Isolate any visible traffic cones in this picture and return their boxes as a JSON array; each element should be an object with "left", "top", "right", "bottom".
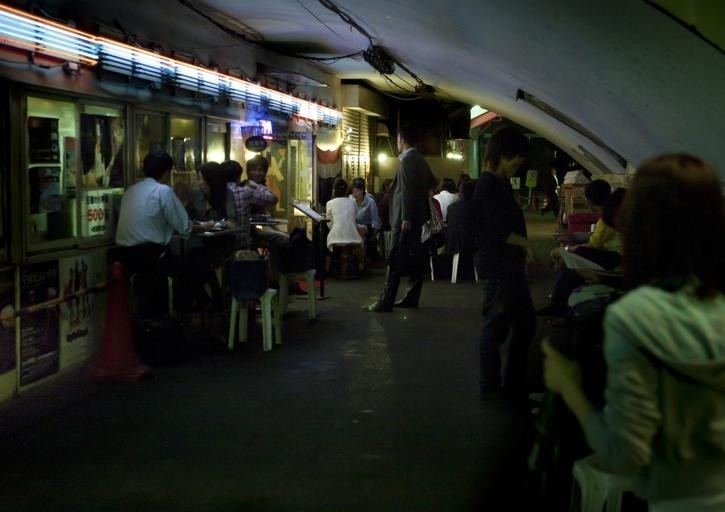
[{"left": 88, "top": 262, "right": 153, "bottom": 384}]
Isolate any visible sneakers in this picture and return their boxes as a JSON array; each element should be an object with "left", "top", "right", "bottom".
[
  {"left": 506, "top": 388, "right": 529, "bottom": 398},
  {"left": 480, "top": 387, "right": 502, "bottom": 397}
]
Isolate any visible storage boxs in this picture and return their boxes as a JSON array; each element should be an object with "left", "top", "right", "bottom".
[{"left": 561, "top": 170, "right": 591, "bottom": 215}]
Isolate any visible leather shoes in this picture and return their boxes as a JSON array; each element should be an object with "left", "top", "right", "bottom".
[
  {"left": 362, "top": 302, "right": 394, "bottom": 312},
  {"left": 394, "top": 299, "right": 418, "bottom": 309}
]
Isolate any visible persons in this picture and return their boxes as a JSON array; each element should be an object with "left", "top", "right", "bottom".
[
  {"left": 535, "top": 167, "right": 627, "bottom": 318},
  {"left": 319, "top": 171, "right": 475, "bottom": 279},
  {"left": 540, "top": 153, "right": 725, "bottom": 512},
  {"left": 367, "top": 124, "right": 437, "bottom": 312},
  {"left": 472, "top": 125, "right": 545, "bottom": 410},
  {"left": 64, "top": 260, "right": 92, "bottom": 328},
  {"left": 115, "top": 150, "right": 307, "bottom": 331}
]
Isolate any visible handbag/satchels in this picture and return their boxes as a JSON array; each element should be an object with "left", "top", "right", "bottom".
[{"left": 225, "top": 251, "right": 269, "bottom": 300}]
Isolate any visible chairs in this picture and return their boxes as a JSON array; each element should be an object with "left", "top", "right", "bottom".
[{"left": 448, "top": 214, "right": 480, "bottom": 284}]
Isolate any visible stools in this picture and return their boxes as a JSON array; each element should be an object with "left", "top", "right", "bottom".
[
  {"left": 570, "top": 453, "right": 635, "bottom": 512},
  {"left": 129, "top": 273, "right": 178, "bottom": 317},
  {"left": 333, "top": 243, "right": 361, "bottom": 280},
  {"left": 227, "top": 287, "right": 282, "bottom": 351},
  {"left": 421, "top": 240, "right": 438, "bottom": 282},
  {"left": 277, "top": 269, "right": 319, "bottom": 320}
]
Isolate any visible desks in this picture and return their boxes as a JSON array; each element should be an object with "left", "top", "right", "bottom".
[
  {"left": 195, "top": 227, "right": 237, "bottom": 329},
  {"left": 248, "top": 220, "right": 289, "bottom": 226}
]
